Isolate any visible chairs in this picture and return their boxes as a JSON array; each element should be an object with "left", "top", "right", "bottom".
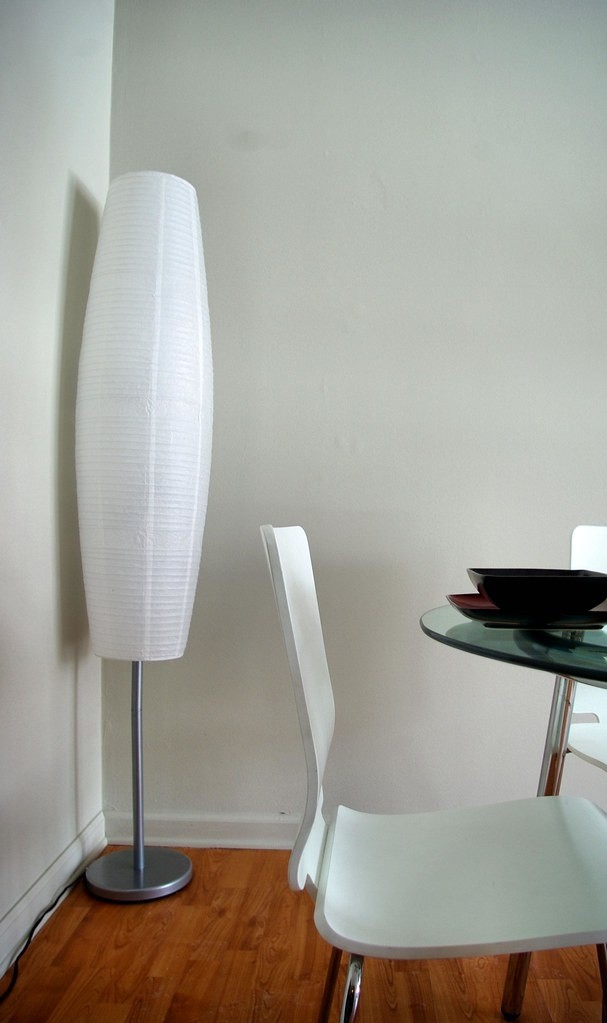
[
  {"left": 563, "top": 524, "right": 607, "bottom": 772},
  {"left": 256, "top": 520, "right": 607, "bottom": 1023}
]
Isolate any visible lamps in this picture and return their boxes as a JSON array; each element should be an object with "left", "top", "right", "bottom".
[{"left": 64, "top": 169, "right": 216, "bottom": 901}]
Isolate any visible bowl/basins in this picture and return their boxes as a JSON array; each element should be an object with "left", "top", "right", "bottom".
[{"left": 465, "top": 567, "right": 607, "bottom": 609}]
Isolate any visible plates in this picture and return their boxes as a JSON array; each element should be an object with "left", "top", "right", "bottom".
[{"left": 446, "top": 593, "right": 607, "bottom": 630}]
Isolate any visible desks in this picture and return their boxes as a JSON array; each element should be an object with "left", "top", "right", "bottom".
[{"left": 418, "top": 604, "right": 607, "bottom": 1023}]
